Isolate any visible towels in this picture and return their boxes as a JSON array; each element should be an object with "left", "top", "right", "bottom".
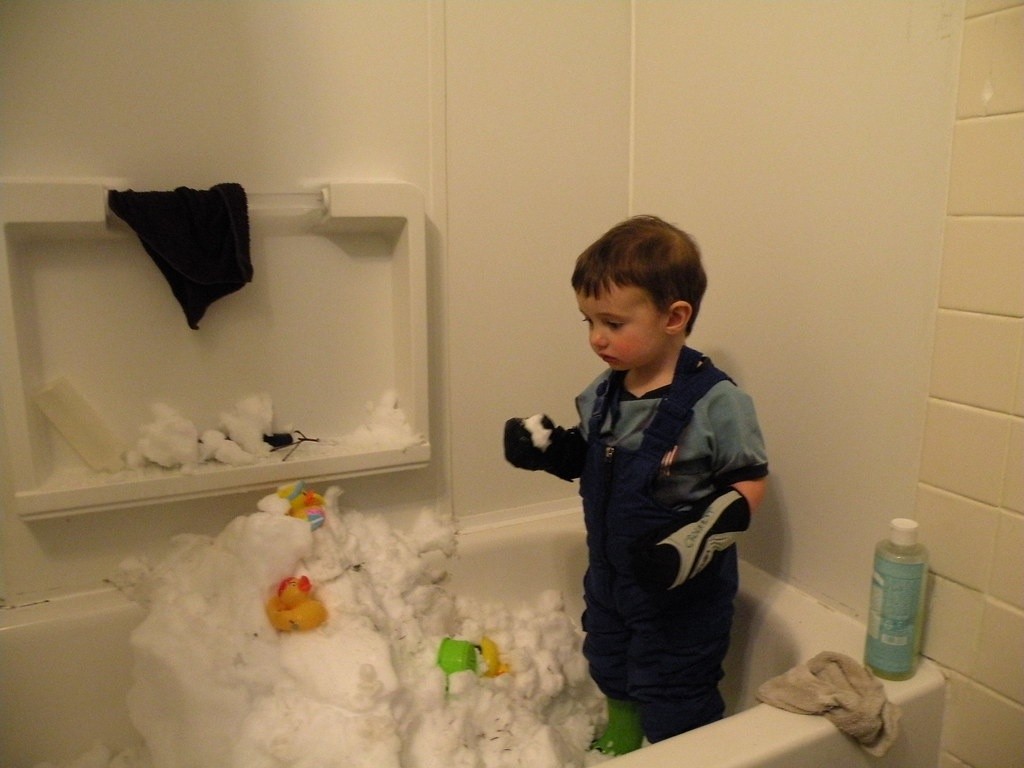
[
  {"left": 106, "top": 180, "right": 254, "bottom": 331},
  {"left": 752, "top": 648, "right": 903, "bottom": 759}
]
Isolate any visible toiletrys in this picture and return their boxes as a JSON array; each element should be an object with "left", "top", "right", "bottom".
[{"left": 862, "top": 516, "right": 933, "bottom": 684}]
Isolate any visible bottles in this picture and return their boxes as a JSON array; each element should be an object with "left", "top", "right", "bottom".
[
  {"left": 32, "top": 375, "right": 127, "bottom": 473},
  {"left": 863, "top": 517, "right": 929, "bottom": 681}
]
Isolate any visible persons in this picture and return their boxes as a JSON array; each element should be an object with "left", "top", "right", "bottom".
[{"left": 503, "top": 215, "right": 772, "bottom": 756}]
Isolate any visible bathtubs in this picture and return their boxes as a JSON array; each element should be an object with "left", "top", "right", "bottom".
[{"left": 0, "top": 491, "right": 951, "bottom": 768}]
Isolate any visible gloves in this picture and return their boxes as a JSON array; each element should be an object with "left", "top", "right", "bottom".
[
  {"left": 633, "top": 487, "right": 752, "bottom": 597},
  {"left": 504, "top": 414, "right": 584, "bottom": 483}
]
